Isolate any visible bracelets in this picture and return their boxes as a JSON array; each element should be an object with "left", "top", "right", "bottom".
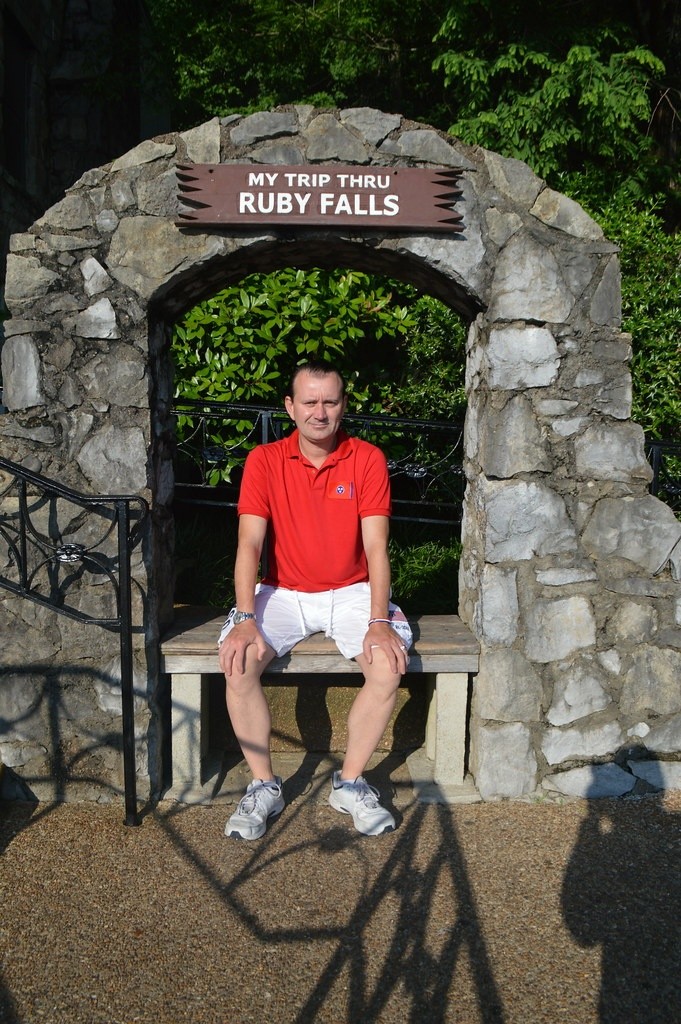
[{"left": 368, "top": 618, "right": 391, "bottom": 626}]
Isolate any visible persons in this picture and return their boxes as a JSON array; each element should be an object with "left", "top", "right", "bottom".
[{"left": 216, "top": 358, "right": 413, "bottom": 842}]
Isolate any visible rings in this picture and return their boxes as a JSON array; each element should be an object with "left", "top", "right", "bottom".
[{"left": 400, "top": 644, "right": 407, "bottom": 651}]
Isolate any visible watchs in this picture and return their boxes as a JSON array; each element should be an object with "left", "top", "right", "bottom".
[{"left": 233, "top": 611, "right": 257, "bottom": 626}]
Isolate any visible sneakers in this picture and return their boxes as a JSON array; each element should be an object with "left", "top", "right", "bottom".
[
  {"left": 224, "top": 776, "right": 285, "bottom": 841},
  {"left": 329, "top": 769, "right": 396, "bottom": 836}
]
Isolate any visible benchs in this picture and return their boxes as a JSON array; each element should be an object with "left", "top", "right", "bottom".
[{"left": 158, "top": 603, "right": 484, "bottom": 806}]
